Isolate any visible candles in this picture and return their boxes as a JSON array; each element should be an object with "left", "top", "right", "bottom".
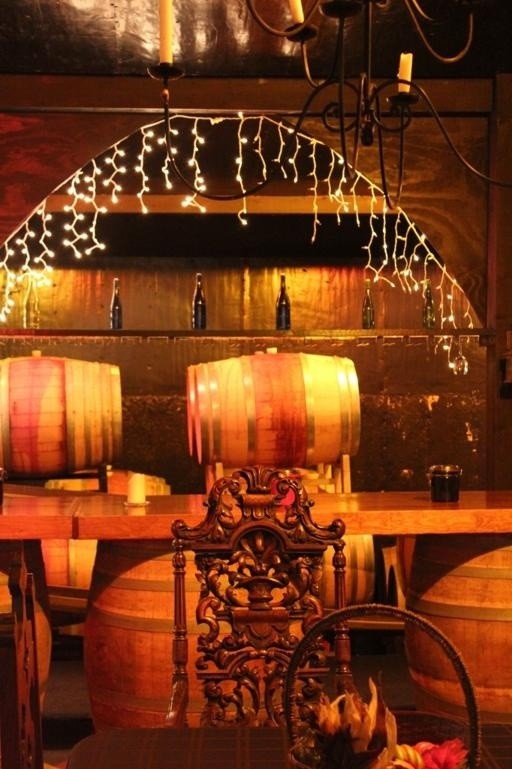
[
  {"left": 397, "top": 52, "right": 414, "bottom": 94},
  {"left": 288, "top": 0, "right": 304, "bottom": 24},
  {"left": 158, "top": 0, "right": 173, "bottom": 67}
]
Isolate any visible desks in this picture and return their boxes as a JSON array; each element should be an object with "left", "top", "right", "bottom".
[{"left": 2, "top": 487, "right": 512, "bottom": 769}]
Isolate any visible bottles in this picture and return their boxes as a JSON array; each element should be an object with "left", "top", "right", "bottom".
[
  {"left": 423, "top": 279, "right": 436, "bottom": 330},
  {"left": 429, "top": 466, "right": 460, "bottom": 501},
  {"left": 110, "top": 279, "right": 122, "bottom": 330},
  {"left": 362, "top": 279, "right": 374, "bottom": 329},
  {"left": 23, "top": 275, "right": 39, "bottom": 329},
  {"left": 192, "top": 274, "right": 206, "bottom": 330},
  {"left": 275, "top": 275, "right": 290, "bottom": 330}
]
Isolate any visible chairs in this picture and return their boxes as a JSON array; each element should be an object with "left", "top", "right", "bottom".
[{"left": 166, "top": 465, "right": 354, "bottom": 731}]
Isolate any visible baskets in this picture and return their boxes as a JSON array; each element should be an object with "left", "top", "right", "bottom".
[{"left": 284, "top": 604, "right": 482, "bottom": 769}]
